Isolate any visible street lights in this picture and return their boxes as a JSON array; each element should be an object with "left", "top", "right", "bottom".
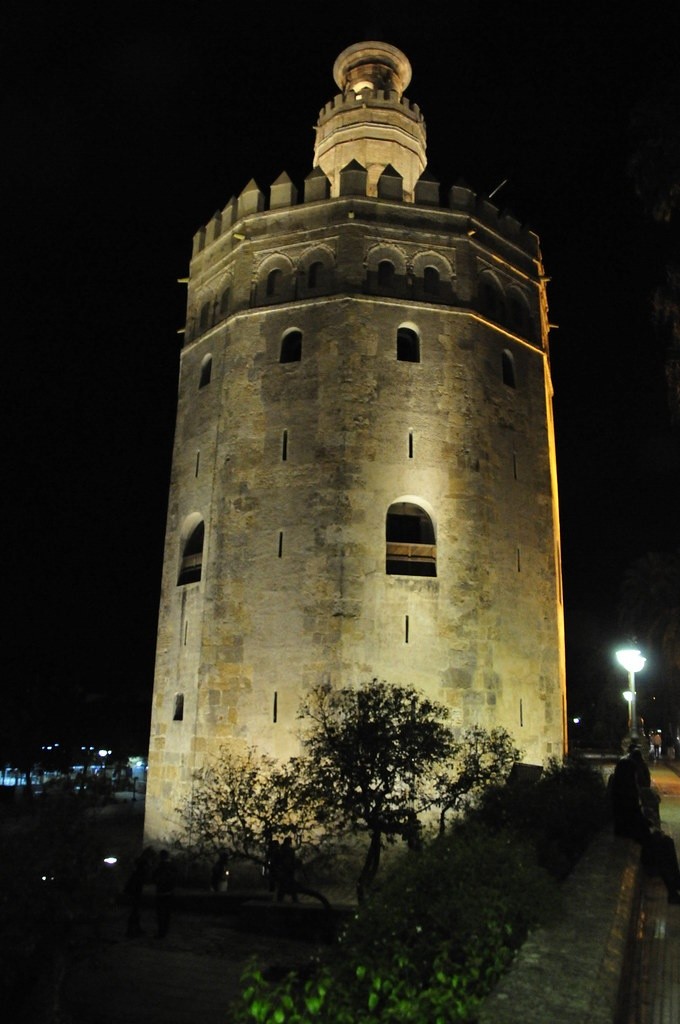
[
  {"left": 80, "top": 746, "right": 95, "bottom": 776},
  {"left": 615, "top": 646, "right": 647, "bottom": 754},
  {"left": 99, "top": 749, "right": 112, "bottom": 778}
]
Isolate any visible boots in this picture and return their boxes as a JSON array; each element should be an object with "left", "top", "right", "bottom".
[{"left": 667, "top": 888, "right": 680, "bottom": 904}]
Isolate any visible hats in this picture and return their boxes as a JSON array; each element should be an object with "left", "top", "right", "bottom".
[{"left": 618, "top": 760, "right": 638, "bottom": 769}]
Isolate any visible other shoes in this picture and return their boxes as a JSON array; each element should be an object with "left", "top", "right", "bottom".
[
  {"left": 123, "top": 926, "right": 146, "bottom": 935},
  {"left": 154, "top": 930, "right": 165, "bottom": 937}
]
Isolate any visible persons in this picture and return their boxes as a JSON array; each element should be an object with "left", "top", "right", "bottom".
[
  {"left": 204, "top": 853, "right": 229, "bottom": 890},
  {"left": 124, "top": 846, "right": 176, "bottom": 939},
  {"left": 266, "top": 837, "right": 302, "bottom": 902},
  {"left": 607, "top": 751, "right": 680, "bottom": 904}
]
